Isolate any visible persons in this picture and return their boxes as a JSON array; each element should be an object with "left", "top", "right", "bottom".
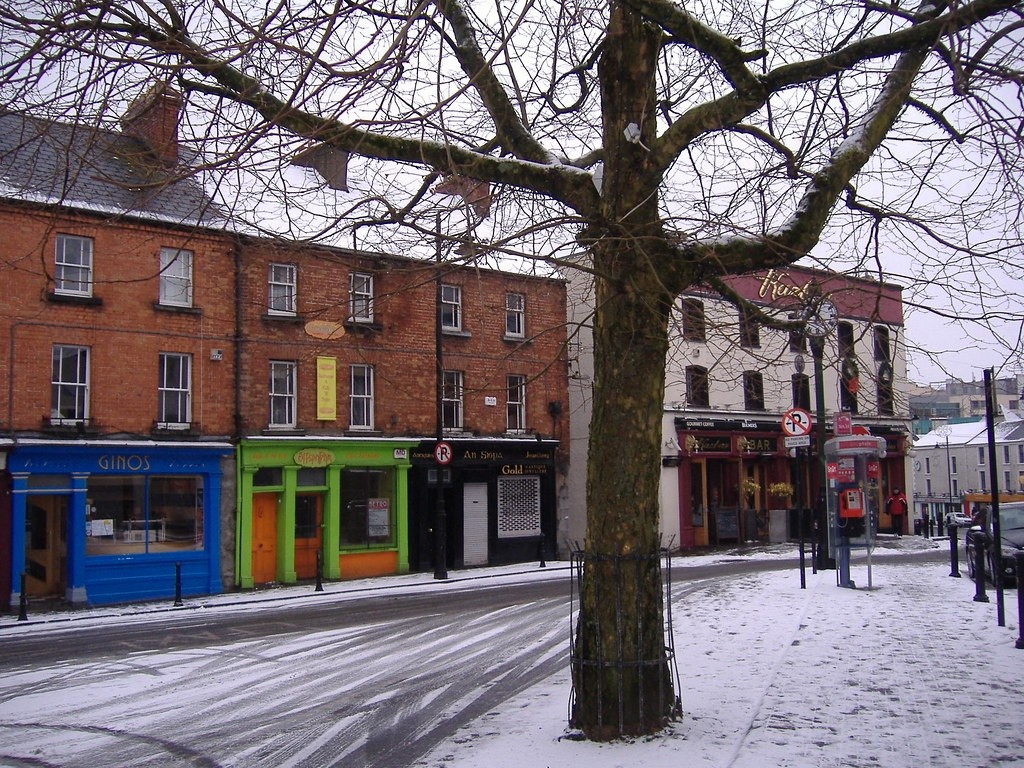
[
  {"left": 885, "top": 486, "right": 908, "bottom": 538},
  {"left": 972, "top": 506, "right": 977, "bottom": 515}
]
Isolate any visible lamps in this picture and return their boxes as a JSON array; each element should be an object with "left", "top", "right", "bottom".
[
  {"left": 746, "top": 442, "right": 751, "bottom": 455},
  {"left": 903, "top": 440, "right": 916, "bottom": 458},
  {"left": 461, "top": 430, "right": 481, "bottom": 438},
  {"left": 784, "top": 440, "right": 796, "bottom": 457},
  {"left": 522, "top": 433, "right": 542, "bottom": 441},
  {"left": 693, "top": 441, "right": 699, "bottom": 453}
]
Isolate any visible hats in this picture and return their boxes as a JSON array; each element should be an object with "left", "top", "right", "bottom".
[{"left": 893, "top": 486, "right": 899, "bottom": 490}]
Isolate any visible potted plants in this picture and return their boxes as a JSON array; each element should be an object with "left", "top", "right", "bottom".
[
  {"left": 768, "top": 484, "right": 792, "bottom": 510},
  {"left": 739, "top": 478, "right": 761, "bottom": 540}
]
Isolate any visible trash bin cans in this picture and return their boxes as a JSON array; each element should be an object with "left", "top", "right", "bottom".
[
  {"left": 947, "top": 523, "right": 956, "bottom": 536},
  {"left": 914, "top": 519, "right": 921, "bottom": 536}
]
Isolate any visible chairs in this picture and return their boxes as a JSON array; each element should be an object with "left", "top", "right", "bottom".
[{"left": 999, "top": 515, "right": 1017, "bottom": 529}]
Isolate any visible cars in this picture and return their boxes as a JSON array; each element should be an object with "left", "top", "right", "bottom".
[
  {"left": 943, "top": 512, "right": 973, "bottom": 528},
  {"left": 965, "top": 501, "right": 1024, "bottom": 587}
]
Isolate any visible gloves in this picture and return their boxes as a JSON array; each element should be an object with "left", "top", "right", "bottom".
[
  {"left": 905, "top": 509, "right": 908, "bottom": 516},
  {"left": 885, "top": 510, "right": 889, "bottom": 515}
]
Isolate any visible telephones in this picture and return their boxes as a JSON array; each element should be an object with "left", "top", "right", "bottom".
[{"left": 839, "top": 489, "right": 863, "bottom": 517}]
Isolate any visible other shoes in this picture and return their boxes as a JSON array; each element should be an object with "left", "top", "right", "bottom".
[
  {"left": 894, "top": 533, "right": 897, "bottom": 537},
  {"left": 898, "top": 535, "right": 902, "bottom": 538}
]
